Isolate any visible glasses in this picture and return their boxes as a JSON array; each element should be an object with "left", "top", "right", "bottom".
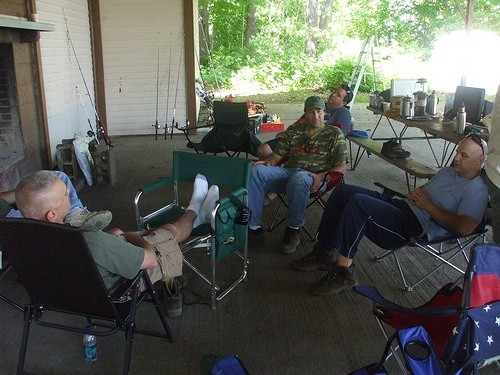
[
  {"left": 340, "top": 84, "right": 351, "bottom": 98},
  {"left": 303, "top": 135, "right": 310, "bottom": 152},
  {"left": 466, "top": 134, "right": 484, "bottom": 155},
  {"left": 55, "top": 184, "right": 70, "bottom": 208}
]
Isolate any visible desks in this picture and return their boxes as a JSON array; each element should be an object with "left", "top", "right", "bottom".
[{"left": 366, "top": 106, "right": 490, "bottom": 168}]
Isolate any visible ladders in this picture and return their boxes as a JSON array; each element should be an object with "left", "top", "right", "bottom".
[{"left": 348, "top": 36, "right": 385, "bottom": 116}]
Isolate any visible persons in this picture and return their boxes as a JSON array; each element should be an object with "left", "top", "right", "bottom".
[
  {"left": 248, "top": 96, "right": 350, "bottom": 254},
  {"left": 0, "top": 171, "right": 219, "bottom": 303},
  {"left": 292, "top": 133, "right": 488, "bottom": 296},
  {"left": 258, "top": 84, "right": 354, "bottom": 208}
]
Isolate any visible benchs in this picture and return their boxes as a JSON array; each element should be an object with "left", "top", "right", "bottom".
[{"left": 346, "top": 134, "right": 437, "bottom": 194}]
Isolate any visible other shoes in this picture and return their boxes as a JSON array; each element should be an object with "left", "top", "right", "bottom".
[{"left": 381, "top": 138, "right": 411, "bottom": 159}]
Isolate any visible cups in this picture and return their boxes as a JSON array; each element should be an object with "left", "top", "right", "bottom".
[{"left": 382, "top": 102, "right": 391, "bottom": 112}]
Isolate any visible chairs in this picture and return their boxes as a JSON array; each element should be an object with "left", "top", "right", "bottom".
[
  {"left": 133, "top": 151, "right": 252, "bottom": 311},
  {"left": 266, "top": 119, "right": 345, "bottom": 243},
  {"left": 185, "top": 101, "right": 263, "bottom": 158},
  {"left": 0, "top": 216, "right": 174, "bottom": 375},
  {"left": 371, "top": 165, "right": 500, "bottom": 293},
  {"left": 352, "top": 242, "right": 500, "bottom": 375}
]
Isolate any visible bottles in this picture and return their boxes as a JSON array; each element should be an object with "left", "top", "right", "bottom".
[
  {"left": 82, "top": 325, "right": 97, "bottom": 362},
  {"left": 349, "top": 117, "right": 354, "bottom": 133},
  {"left": 400, "top": 92, "right": 427, "bottom": 116}
]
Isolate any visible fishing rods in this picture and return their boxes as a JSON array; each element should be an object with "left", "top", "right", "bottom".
[
  {"left": 162, "top": 32, "right": 171, "bottom": 139},
  {"left": 170, "top": 31, "right": 184, "bottom": 140},
  {"left": 62, "top": 8, "right": 112, "bottom": 147},
  {"left": 76, "top": 85, "right": 100, "bottom": 145},
  {"left": 152, "top": 32, "right": 161, "bottom": 140}
]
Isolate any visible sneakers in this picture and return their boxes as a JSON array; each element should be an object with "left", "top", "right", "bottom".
[
  {"left": 279, "top": 226, "right": 301, "bottom": 253},
  {"left": 309, "top": 260, "right": 359, "bottom": 296},
  {"left": 248, "top": 226, "right": 265, "bottom": 244},
  {"left": 291, "top": 243, "right": 337, "bottom": 272}
]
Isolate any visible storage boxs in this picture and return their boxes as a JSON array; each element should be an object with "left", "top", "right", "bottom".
[
  {"left": 247, "top": 113, "right": 268, "bottom": 135},
  {"left": 262, "top": 120, "right": 284, "bottom": 131}
]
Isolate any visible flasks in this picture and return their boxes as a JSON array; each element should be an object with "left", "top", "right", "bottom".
[
  {"left": 430, "top": 90, "right": 437, "bottom": 115},
  {"left": 458, "top": 106, "right": 466, "bottom": 135}
]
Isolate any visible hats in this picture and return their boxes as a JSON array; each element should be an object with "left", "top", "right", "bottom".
[{"left": 305, "top": 96, "right": 325, "bottom": 110}]
